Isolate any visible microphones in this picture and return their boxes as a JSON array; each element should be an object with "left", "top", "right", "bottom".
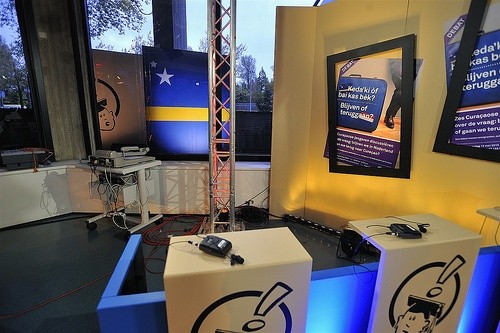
[
  {"left": 230, "top": 254, "right": 244, "bottom": 265},
  {"left": 418, "top": 225, "right": 427, "bottom": 233}
]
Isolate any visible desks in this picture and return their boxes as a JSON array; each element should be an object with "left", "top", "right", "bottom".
[
  {"left": 75, "top": 160, "right": 164, "bottom": 242},
  {"left": 97, "top": 206, "right": 500, "bottom": 333}
]
